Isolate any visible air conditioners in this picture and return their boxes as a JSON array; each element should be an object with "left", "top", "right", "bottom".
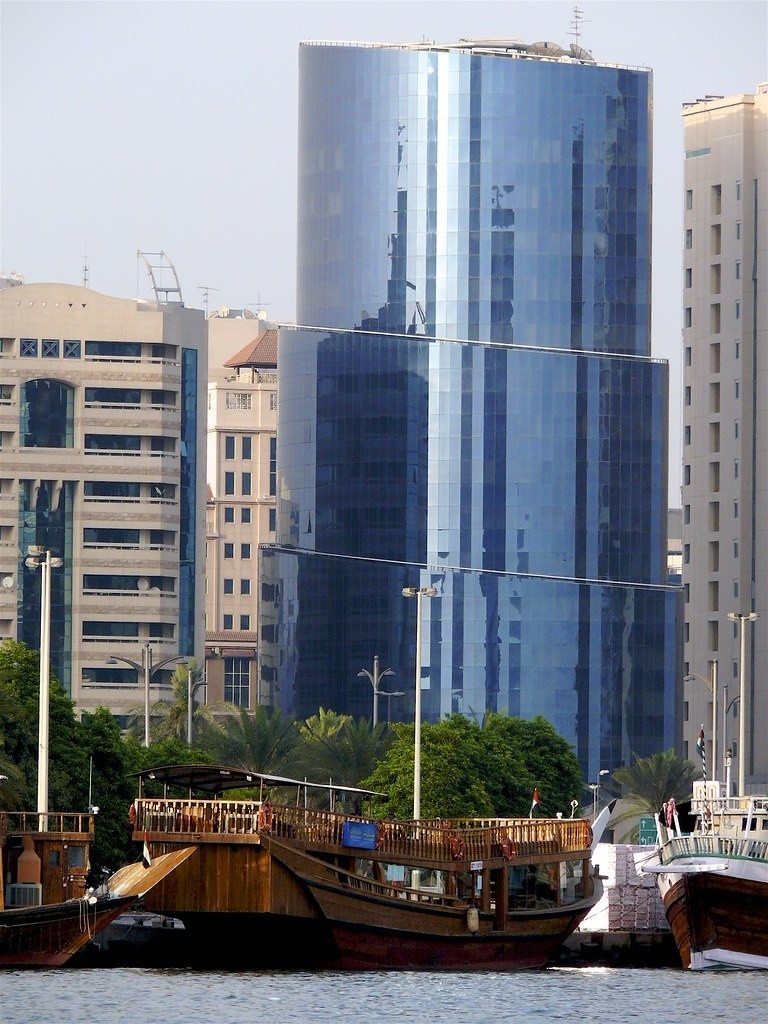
[{"left": 5, "top": 883, "right": 42, "bottom": 909}]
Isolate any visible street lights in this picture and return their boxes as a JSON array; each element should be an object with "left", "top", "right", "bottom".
[
  {"left": 726, "top": 611, "right": 761, "bottom": 798},
  {"left": 374, "top": 691, "right": 405, "bottom": 724},
  {"left": 357, "top": 655, "right": 398, "bottom": 729},
  {"left": 402, "top": 586, "right": 438, "bottom": 838},
  {"left": 24, "top": 544, "right": 64, "bottom": 833},
  {"left": 105, "top": 642, "right": 190, "bottom": 749},
  {"left": 684, "top": 658, "right": 720, "bottom": 781}
]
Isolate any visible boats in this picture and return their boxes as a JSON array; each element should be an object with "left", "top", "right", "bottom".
[
  {"left": 0, "top": 801, "right": 106, "bottom": 973},
  {"left": 131, "top": 788, "right": 605, "bottom": 976},
  {"left": 641, "top": 722, "right": 768, "bottom": 977}
]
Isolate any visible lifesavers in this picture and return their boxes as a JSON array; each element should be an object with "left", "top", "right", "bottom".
[
  {"left": 373, "top": 822, "right": 386, "bottom": 849},
  {"left": 129, "top": 803, "right": 135, "bottom": 826},
  {"left": 441, "top": 818, "right": 453, "bottom": 837},
  {"left": 502, "top": 837, "right": 516, "bottom": 861},
  {"left": 451, "top": 837, "right": 465, "bottom": 860},
  {"left": 583, "top": 825, "right": 593, "bottom": 849},
  {"left": 259, "top": 805, "right": 272, "bottom": 834}
]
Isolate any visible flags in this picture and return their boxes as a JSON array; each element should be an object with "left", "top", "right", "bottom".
[
  {"left": 529, "top": 788, "right": 541, "bottom": 819},
  {"left": 696, "top": 724, "right": 705, "bottom": 759},
  {"left": 142, "top": 828, "right": 151, "bottom": 869}
]
